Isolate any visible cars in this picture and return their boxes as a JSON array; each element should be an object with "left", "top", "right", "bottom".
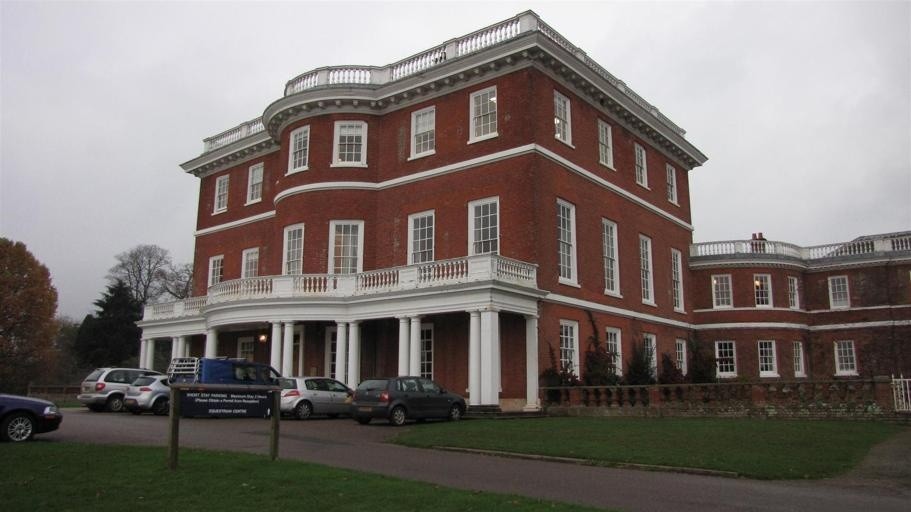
[{"left": 0, "top": 394, "right": 61, "bottom": 441}]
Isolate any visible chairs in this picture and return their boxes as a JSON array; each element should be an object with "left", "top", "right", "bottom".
[{"left": 406, "top": 382, "right": 416, "bottom": 390}]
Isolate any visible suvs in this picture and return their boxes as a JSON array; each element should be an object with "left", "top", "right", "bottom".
[
  {"left": 351, "top": 376, "right": 466, "bottom": 425},
  {"left": 275, "top": 377, "right": 351, "bottom": 420},
  {"left": 167, "top": 354, "right": 283, "bottom": 384},
  {"left": 77, "top": 367, "right": 171, "bottom": 416}
]
